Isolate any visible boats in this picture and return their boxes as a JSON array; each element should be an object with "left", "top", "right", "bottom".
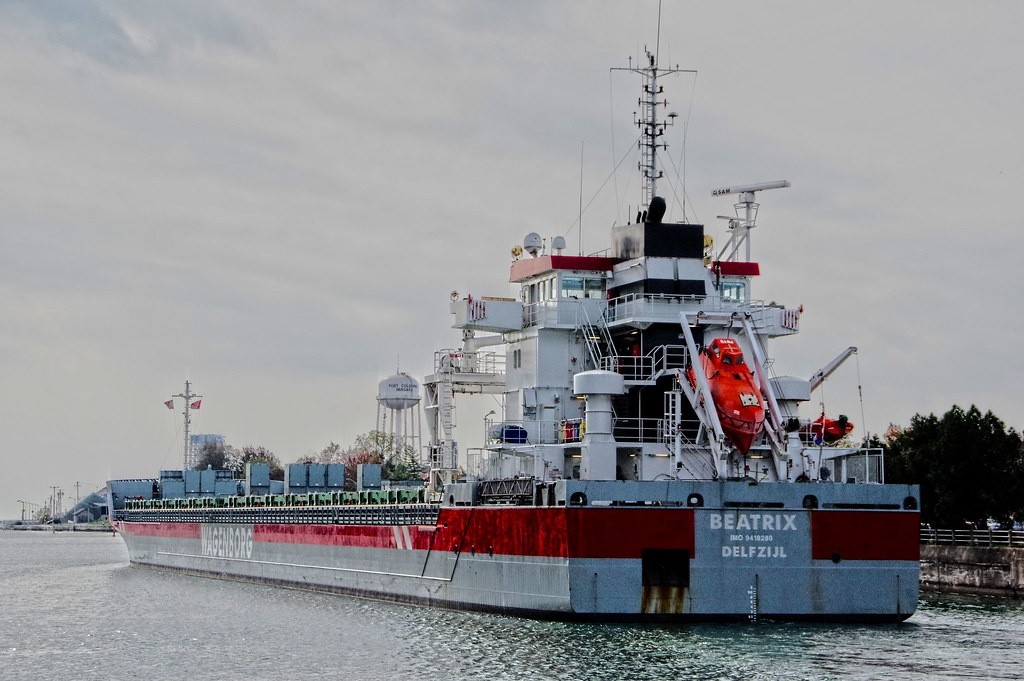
[
  {"left": 800, "top": 413, "right": 855, "bottom": 442},
  {"left": 11, "top": 523, "right": 115, "bottom": 530},
  {"left": 685, "top": 338, "right": 765, "bottom": 457},
  {"left": 107, "top": 0, "right": 921, "bottom": 620}
]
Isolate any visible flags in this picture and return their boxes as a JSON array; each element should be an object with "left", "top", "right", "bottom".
[
  {"left": 191, "top": 400, "right": 201, "bottom": 408},
  {"left": 165, "top": 400, "right": 174, "bottom": 409}
]
